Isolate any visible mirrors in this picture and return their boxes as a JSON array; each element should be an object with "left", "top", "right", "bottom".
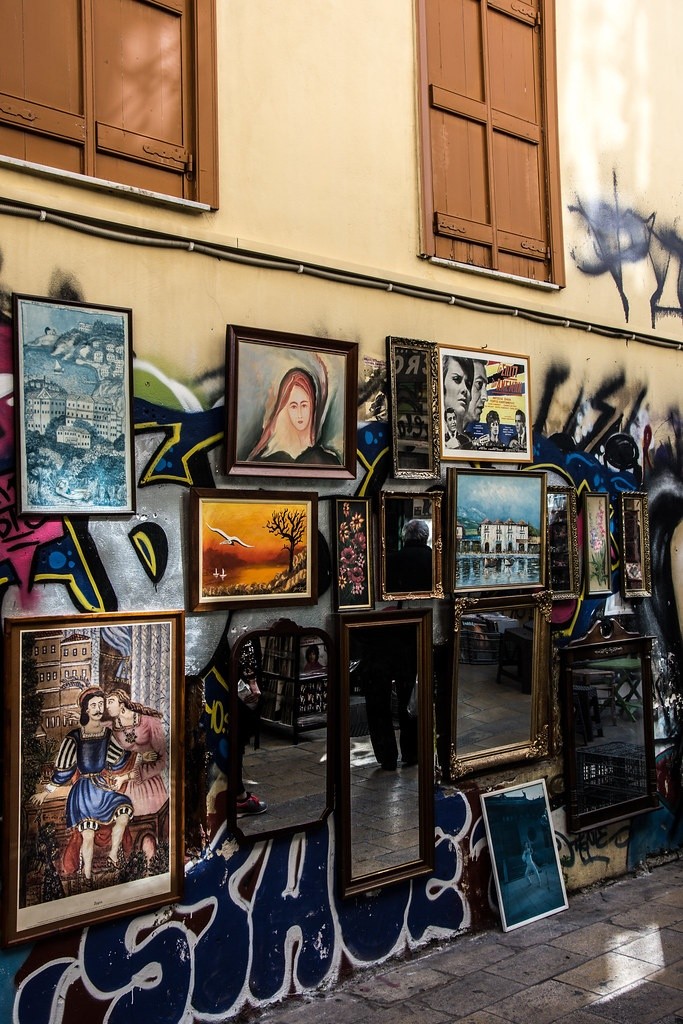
[
  {"left": 547, "top": 485, "right": 581, "bottom": 601},
  {"left": 559, "top": 618, "right": 664, "bottom": 837},
  {"left": 449, "top": 589, "right": 554, "bottom": 782},
  {"left": 385, "top": 335, "right": 442, "bottom": 481},
  {"left": 228, "top": 616, "right": 334, "bottom": 845},
  {"left": 333, "top": 608, "right": 435, "bottom": 901},
  {"left": 379, "top": 490, "right": 445, "bottom": 601}
]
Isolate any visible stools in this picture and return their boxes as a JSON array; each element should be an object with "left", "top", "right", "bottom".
[
  {"left": 572, "top": 684, "right": 603, "bottom": 741},
  {"left": 588, "top": 659, "right": 641, "bottom": 723},
  {"left": 572, "top": 668, "right": 617, "bottom": 727}
]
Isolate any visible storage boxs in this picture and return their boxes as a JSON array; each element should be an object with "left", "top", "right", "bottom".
[{"left": 575, "top": 739, "right": 646, "bottom": 815}]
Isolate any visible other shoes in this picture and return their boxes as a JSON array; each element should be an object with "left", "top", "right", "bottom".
[
  {"left": 381, "top": 760, "right": 397, "bottom": 771},
  {"left": 401, "top": 754, "right": 417, "bottom": 764}
]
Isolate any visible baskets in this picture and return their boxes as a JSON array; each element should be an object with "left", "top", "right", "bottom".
[
  {"left": 459, "top": 629, "right": 501, "bottom": 665},
  {"left": 576, "top": 740, "right": 648, "bottom": 814}
]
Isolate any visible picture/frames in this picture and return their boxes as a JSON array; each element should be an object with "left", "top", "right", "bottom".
[
  {"left": 436, "top": 342, "right": 534, "bottom": 464},
  {"left": 584, "top": 491, "right": 612, "bottom": 597},
  {"left": 226, "top": 323, "right": 359, "bottom": 479},
  {"left": 480, "top": 778, "right": 569, "bottom": 933},
  {"left": 330, "top": 494, "right": 376, "bottom": 612},
  {"left": 11, "top": 291, "right": 136, "bottom": 516},
  {"left": 188, "top": 486, "right": 318, "bottom": 613},
  {"left": 618, "top": 491, "right": 652, "bottom": 599},
  {"left": 0, "top": 609, "right": 188, "bottom": 949},
  {"left": 445, "top": 465, "right": 545, "bottom": 593}
]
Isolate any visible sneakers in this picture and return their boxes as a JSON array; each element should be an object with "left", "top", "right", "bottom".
[{"left": 237, "top": 791, "right": 269, "bottom": 818}]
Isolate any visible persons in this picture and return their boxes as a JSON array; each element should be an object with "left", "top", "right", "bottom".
[
  {"left": 353, "top": 623, "right": 419, "bottom": 769},
  {"left": 388, "top": 519, "right": 432, "bottom": 590},
  {"left": 443, "top": 356, "right": 526, "bottom": 453},
  {"left": 234, "top": 637, "right": 268, "bottom": 818},
  {"left": 521, "top": 841, "right": 542, "bottom": 887}
]
